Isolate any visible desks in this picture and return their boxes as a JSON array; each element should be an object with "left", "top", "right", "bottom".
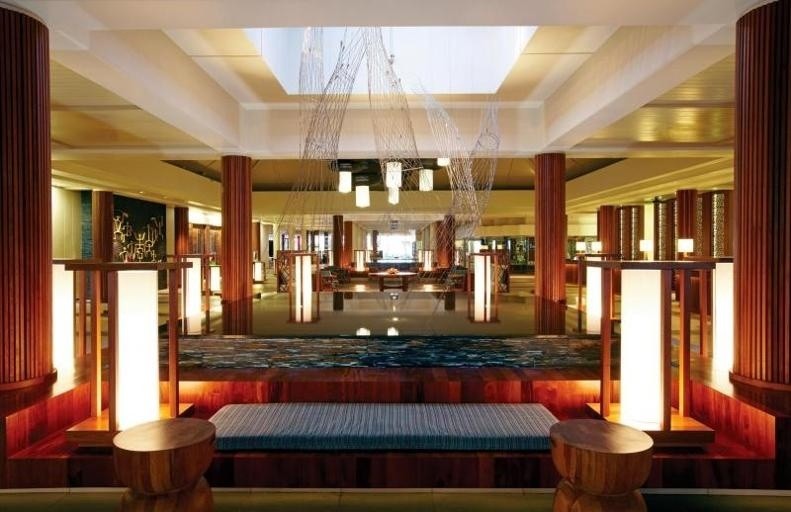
[{"left": 368, "top": 272, "right": 419, "bottom": 292}]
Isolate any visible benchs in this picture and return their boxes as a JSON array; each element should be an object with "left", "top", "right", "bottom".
[{"left": 204, "top": 402, "right": 560, "bottom": 486}]
